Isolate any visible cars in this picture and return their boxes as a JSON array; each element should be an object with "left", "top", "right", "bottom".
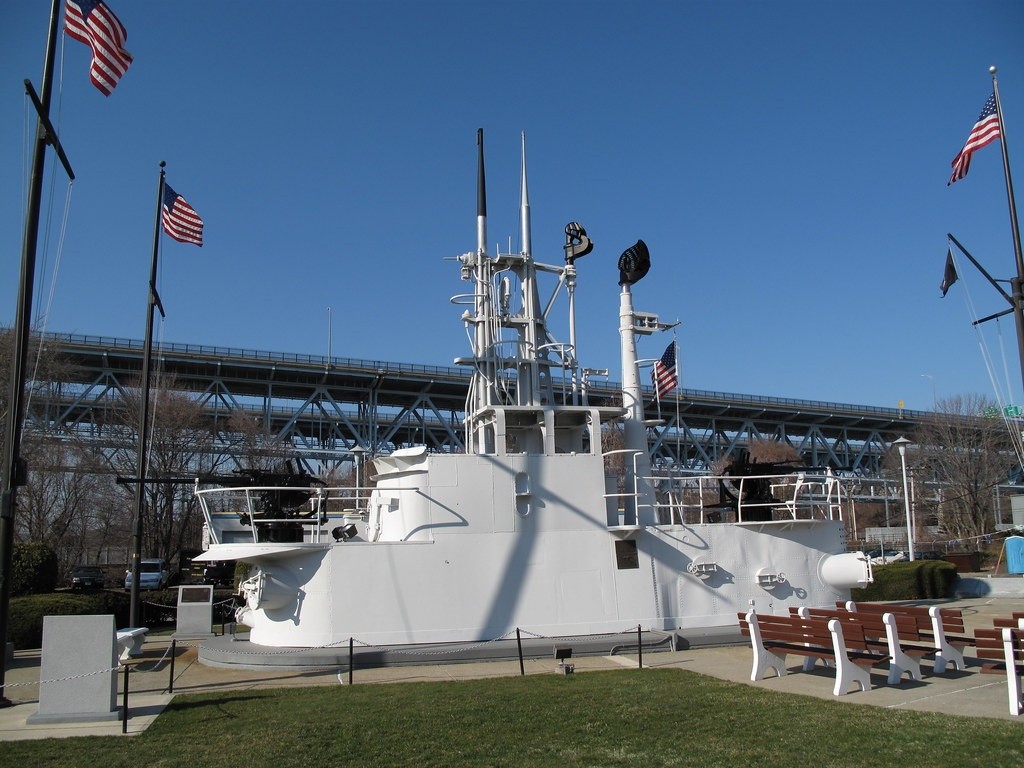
[{"left": 72, "top": 565, "right": 106, "bottom": 591}]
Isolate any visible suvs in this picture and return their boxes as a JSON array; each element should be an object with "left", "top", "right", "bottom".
[{"left": 125, "top": 558, "right": 169, "bottom": 592}]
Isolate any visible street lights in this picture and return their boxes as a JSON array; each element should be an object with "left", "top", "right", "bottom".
[
  {"left": 892, "top": 436, "right": 914, "bottom": 562},
  {"left": 921, "top": 374, "right": 937, "bottom": 423}
]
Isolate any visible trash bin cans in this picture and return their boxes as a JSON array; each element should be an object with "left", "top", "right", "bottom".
[{"left": 1005, "top": 536, "right": 1024, "bottom": 575}]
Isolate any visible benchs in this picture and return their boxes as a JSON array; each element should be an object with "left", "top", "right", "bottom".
[
  {"left": 117, "top": 627, "right": 149, "bottom": 666},
  {"left": 736, "top": 600, "right": 1024, "bottom": 716}
]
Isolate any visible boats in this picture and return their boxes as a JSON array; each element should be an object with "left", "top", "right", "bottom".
[{"left": 192, "top": 127, "right": 873, "bottom": 670}]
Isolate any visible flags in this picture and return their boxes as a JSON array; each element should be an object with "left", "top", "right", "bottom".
[
  {"left": 162, "top": 182, "right": 204, "bottom": 248},
  {"left": 650, "top": 342, "right": 678, "bottom": 402},
  {"left": 947, "top": 91, "right": 1001, "bottom": 186},
  {"left": 940, "top": 250, "right": 958, "bottom": 298},
  {"left": 64, "top": 0, "right": 135, "bottom": 97}
]
